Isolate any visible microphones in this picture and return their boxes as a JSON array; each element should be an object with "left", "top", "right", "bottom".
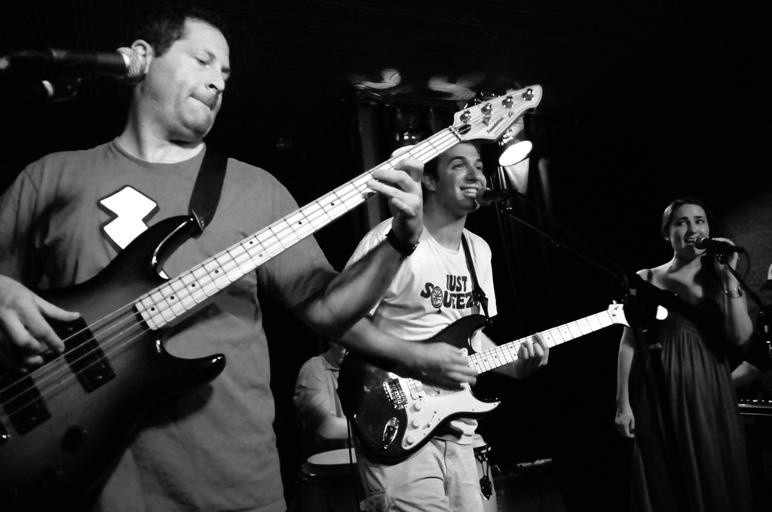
[
  {"left": 44, "top": 45, "right": 148, "bottom": 86},
  {"left": 475, "top": 187, "right": 509, "bottom": 206},
  {"left": 693, "top": 233, "right": 746, "bottom": 255}
]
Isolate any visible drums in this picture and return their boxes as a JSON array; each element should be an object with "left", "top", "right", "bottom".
[{"left": 298, "top": 447, "right": 366, "bottom": 512}]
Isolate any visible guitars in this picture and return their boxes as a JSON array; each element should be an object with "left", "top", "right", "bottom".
[
  {"left": 339, "top": 299, "right": 631, "bottom": 464},
  {"left": 0, "top": 83, "right": 544, "bottom": 512}
]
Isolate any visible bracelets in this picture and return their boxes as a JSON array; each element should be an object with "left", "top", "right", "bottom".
[
  {"left": 385, "top": 227, "right": 419, "bottom": 258},
  {"left": 723, "top": 286, "right": 745, "bottom": 301}
]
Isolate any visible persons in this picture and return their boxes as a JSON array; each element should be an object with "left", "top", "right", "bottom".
[
  {"left": 328, "top": 138, "right": 551, "bottom": 512},
  {"left": 614, "top": 195, "right": 761, "bottom": 510},
  {"left": 0, "top": 6, "right": 424, "bottom": 512},
  {"left": 291, "top": 325, "right": 355, "bottom": 442}
]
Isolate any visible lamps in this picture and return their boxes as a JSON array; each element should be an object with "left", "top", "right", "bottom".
[{"left": 498, "top": 137, "right": 533, "bottom": 167}]
